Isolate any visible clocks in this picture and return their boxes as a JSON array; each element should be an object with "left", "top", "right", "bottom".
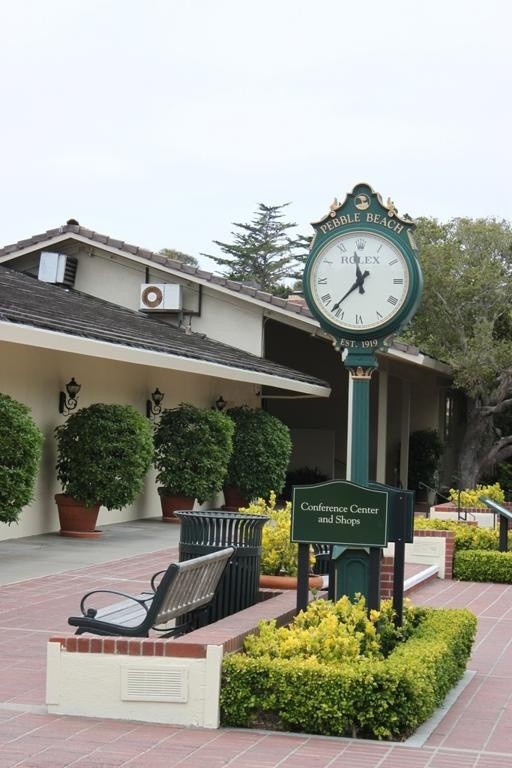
[{"left": 309, "top": 231, "right": 410, "bottom": 331}]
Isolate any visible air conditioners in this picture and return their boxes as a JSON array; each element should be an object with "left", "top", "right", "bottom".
[{"left": 139, "top": 283, "right": 183, "bottom": 312}]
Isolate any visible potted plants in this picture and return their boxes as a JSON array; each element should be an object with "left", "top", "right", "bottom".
[
  {"left": 53, "top": 403, "right": 155, "bottom": 531},
  {"left": 152, "top": 402, "right": 237, "bottom": 517},
  {"left": 223, "top": 403, "right": 294, "bottom": 507}
]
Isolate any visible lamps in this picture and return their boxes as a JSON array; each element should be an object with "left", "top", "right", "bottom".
[{"left": 59, "top": 377, "right": 227, "bottom": 421}]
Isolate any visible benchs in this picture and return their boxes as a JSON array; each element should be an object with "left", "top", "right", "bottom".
[{"left": 68, "top": 546, "right": 238, "bottom": 639}]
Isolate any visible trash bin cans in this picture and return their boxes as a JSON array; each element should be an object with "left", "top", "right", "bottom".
[{"left": 171, "top": 509, "right": 271, "bottom": 639}]
